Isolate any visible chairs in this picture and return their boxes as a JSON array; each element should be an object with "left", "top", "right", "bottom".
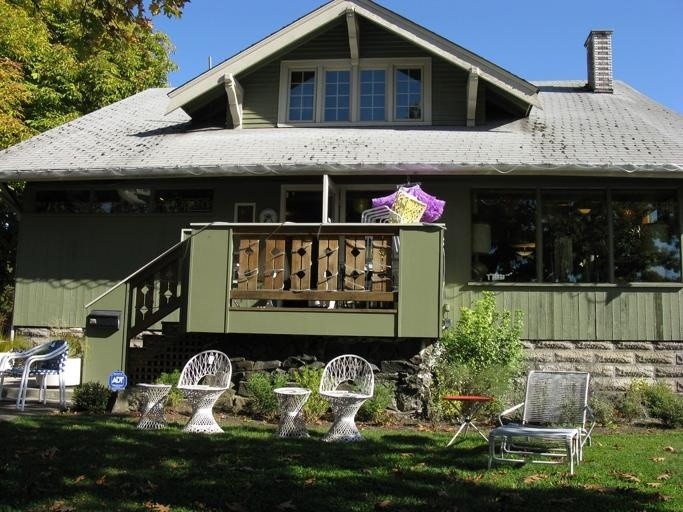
[
  {"left": 358, "top": 190, "right": 426, "bottom": 255},
  {"left": 176, "top": 350, "right": 233, "bottom": 433},
  {"left": 0, "top": 339, "right": 72, "bottom": 413},
  {"left": 486, "top": 368, "right": 597, "bottom": 474},
  {"left": 317, "top": 353, "right": 374, "bottom": 443}
]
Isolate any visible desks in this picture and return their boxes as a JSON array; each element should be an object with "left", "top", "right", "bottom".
[{"left": 440, "top": 390, "right": 491, "bottom": 447}]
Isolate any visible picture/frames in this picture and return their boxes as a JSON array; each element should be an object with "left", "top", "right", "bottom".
[{"left": 233, "top": 201, "right": 257, "bottom": 223}]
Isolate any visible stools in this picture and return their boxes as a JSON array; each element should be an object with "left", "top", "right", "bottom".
[
  {"left": 135, "top": 380, "right": 172, "bottom": 430},
  {"left": 273, "top": 384, "right": 310, "bottom": 441}
]
